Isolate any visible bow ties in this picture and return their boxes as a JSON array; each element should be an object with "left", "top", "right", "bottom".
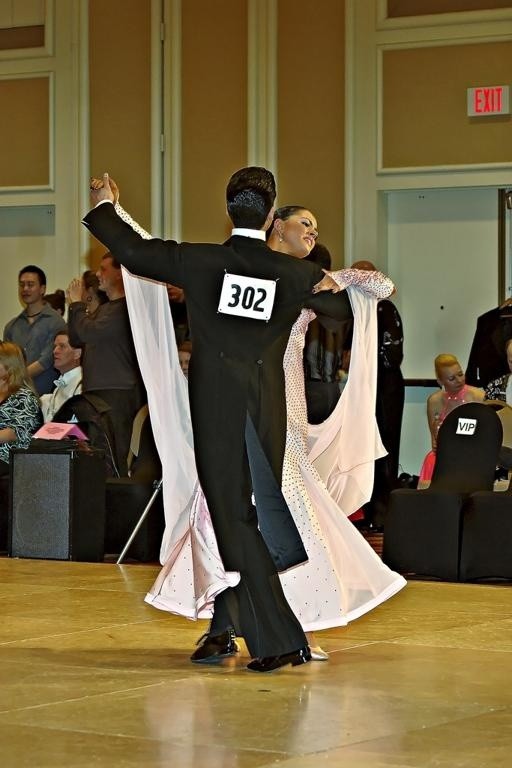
[{"left": 52, "top": 378, "right": 65, "bottom": 386}]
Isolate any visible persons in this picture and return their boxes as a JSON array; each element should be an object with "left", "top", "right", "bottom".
[
  {"left": 79, "top": 164, "right": 354, "bottom": 671},
  {"left": 425, "top": 352, "right": 485, "bottom": 455},
  {"left": 89, "top": 173, "right": 400, "bottom": 661},
  {"left": 483, "top": 337, "right": 512, "bottom": 407},
  {"left": 1, "top": 250, "right": 405, "bottom": 533}
]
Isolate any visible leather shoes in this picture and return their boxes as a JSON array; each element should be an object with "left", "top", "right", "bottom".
[
  {"left": 247, "top": 646, "right": 312, "bottom": 671},
  {"left": 191, "top": 637, "right": 234, "bottom": 663},
  {"left": 310, "top": 645, "right": 329, "bottom": 659}
]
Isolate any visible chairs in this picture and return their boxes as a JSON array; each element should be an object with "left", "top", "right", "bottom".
[{"left": 382, "top": 402, "right": 504, "bottom": 583}]
